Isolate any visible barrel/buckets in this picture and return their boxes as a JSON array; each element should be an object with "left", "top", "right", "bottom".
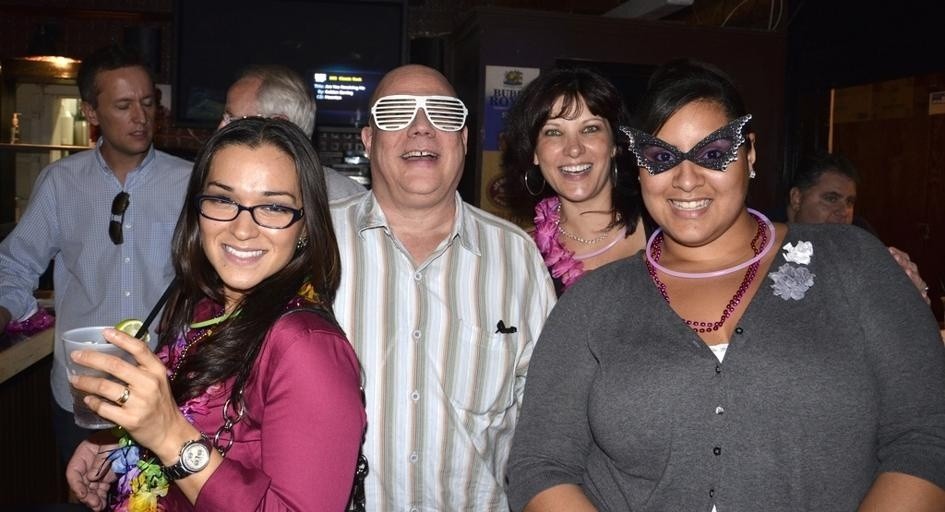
[{"left": 72, "top": 120, "right": 90, "bottom": 147}]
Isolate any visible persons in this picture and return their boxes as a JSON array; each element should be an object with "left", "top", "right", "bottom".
[
  {"left": 216, "top": 64, "right": 368, "bottom": 207},
  {"left": 0, "top": 45, "right": 196, "bottom": 480},
  {"left": 763, "top": 148, "right": 931, "bottom": 317},
  {"left": 64, "top": 116, "right": 368, "bottom": 512},
  {"left": 506, "top": 57, "right": 945, "bottom": 512},
  {"left": 326, "top": 61, "right": 560, "bottom": 512},
  {"left": 498, "top": 66, "right": 658, "bottom": 303}
]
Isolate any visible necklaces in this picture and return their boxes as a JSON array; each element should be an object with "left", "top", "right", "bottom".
[
  {"left": 644, "top": 209, "right": 767, "bottom": 336},
  {"left": 555, "top": 199, "right": 620, "bottom": 246}
]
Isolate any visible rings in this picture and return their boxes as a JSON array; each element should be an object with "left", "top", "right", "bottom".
[
  {"left": 923, "top": 286, "right": 929, "bottom": 291},
  {"left": 117, "top": 385, "right": 130, "bottom": 406}
]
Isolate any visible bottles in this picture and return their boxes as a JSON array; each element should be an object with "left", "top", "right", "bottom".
[
  {"left": 59, "top": 109, "right": 75, "bottom": 148},
  {"left": 30, "top": 156, "right": 40, "bottom": 190}
]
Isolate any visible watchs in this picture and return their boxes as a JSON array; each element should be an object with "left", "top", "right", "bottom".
[{"left": 160, "top": 429, "right": 213, "bottom": 482}]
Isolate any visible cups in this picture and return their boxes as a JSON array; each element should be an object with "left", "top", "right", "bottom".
[
  {"left": 74, "top": 120, "right": 88, "bottom": 146},
  {"left": 58, "top": 325, "right": 150, "bottom": 430}
]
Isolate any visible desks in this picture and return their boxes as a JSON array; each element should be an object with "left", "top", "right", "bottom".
[{"left": 1, "top": 299, "right": 56, "bottom": 382}]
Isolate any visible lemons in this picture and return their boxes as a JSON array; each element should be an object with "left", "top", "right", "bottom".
[{"left": 115, "top": 318, "right": 151, "bottom": 343}]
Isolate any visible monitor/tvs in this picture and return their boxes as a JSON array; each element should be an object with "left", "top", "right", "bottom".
[{"left": 308, "top": 65, "right": 384, "bottom": 126}]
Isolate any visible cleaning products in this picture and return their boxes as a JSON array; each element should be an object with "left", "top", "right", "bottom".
[{"left": 9, "top": 112, "right": 23, "bottom": 144}]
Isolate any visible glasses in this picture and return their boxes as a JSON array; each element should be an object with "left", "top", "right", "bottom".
[
  {"left": 109, "top": 192, "right": 130, "bottom": 244},
  {"left": 370, "top": 94, "right": 468, "bottom": 133},
  {"left": 199, "top": 195, "right": 305, "bottom": 229}
]
